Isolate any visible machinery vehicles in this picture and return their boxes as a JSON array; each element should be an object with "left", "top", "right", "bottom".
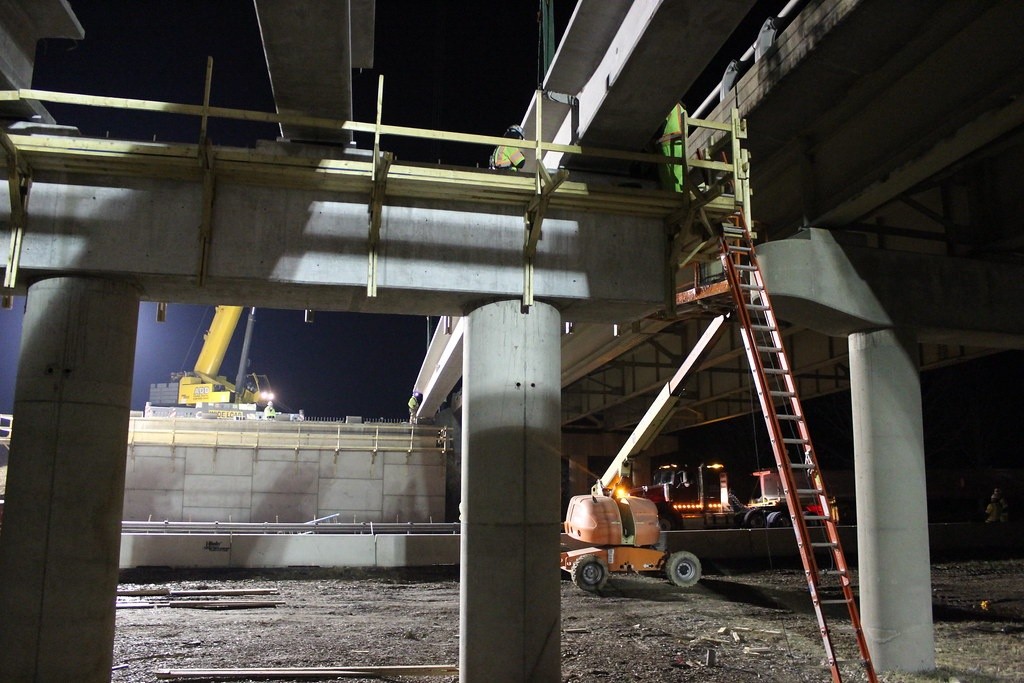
[
  {"left": 557, "top": 305, "right": 839, "bottom": 591},
  {"left": 138, "top": 303, "right": 307, "bottom": 420}
]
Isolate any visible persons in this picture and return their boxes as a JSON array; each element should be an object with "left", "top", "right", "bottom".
[
  {"left": 489, "top": 124, "right": 526, "bottom": 172},
  {"left": 984, "top": 488, "right": 1008, "bottom": 523},
  {"left": 410, "top": 405, "right": 417, "bottom": 424},
  {"left": 659, "top": 103, "right": 685, "bottom": 193},
  {"left": 264, "top": 401, "right": 275, "bottom": 418}
]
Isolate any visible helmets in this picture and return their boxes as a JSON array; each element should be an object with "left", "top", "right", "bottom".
[{"left": 267, "top": 401, "right": 273, "bottom": 406}]
